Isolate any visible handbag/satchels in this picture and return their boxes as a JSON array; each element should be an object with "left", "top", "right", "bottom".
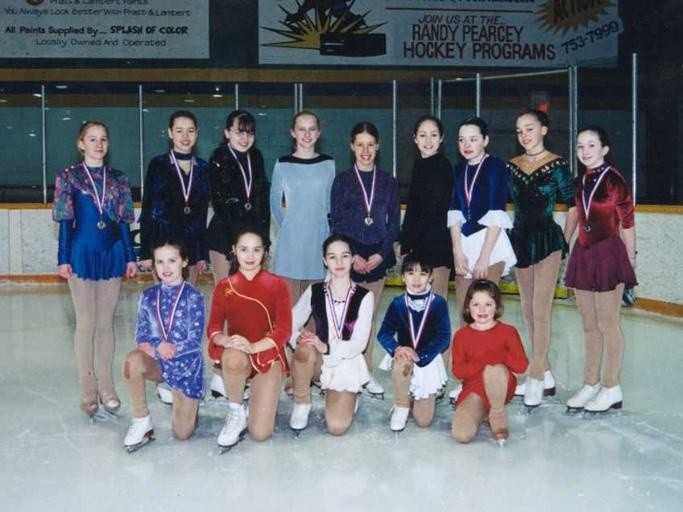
[{"left": 629, "top": 255, "right": 636, "bottom": 261}]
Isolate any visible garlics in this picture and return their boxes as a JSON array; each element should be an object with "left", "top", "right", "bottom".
[
  {"left": 243, "top": 201, "right": 254, "bottom": 212},
  {"left": 465, "top": 208, "right": 473, "bottom": 220},
  {"left": 182, "top": 205, "right": 193, "bottom": 216},
  {"left": 584, "top": 221, "right": 594, "bottom": 232},
  {"left": 94, "top": 217, "right": 108, "bottom": 232},
  {"left": 362, "top": 216, "right": 375, "bottom": 227}
]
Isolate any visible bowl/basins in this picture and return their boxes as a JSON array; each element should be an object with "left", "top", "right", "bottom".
[{"left": 525, "top": 148, "right": 548, "bottom": 157}]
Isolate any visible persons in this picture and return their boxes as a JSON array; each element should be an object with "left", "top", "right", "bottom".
[
  {"left": 504, "top": 102, "right": 580, "bottom": 406},
  {"left": 207, "top": 230, "right": 293, "bottom": 446},
  {"left": 291, "top": 233, "right": 374, "bottom": 435},
  {"left": 376, "top": 252, "right": 452, "bottom": 430},
  {"left": 138, "top": 110, "right": 208, "bottom": 404},
  {"left": 400, "top": 115, "right": 455, "bottom": 401},
  {"left": 51, "top": 121, "right": 138, "bottom": 415},
  {"left": 562, "top": 124, "right": 639, "bottom": 411},
  {"left": 207, "top": 111, "right": 269, "bottom": 402},
  {"left": 446, "top": 117, "right": 519, "bottom": 405},
  {"left": 331, "top": 120, "right": 402, "bottom": 396},
  {"left": 123, "top": 232, "right": 207, "bottom": 446},
  {"left": 270, "top": 110, "right": 335, "bottom": 398},
  {"left": 451, "top": 277, "right": 529, "bottom": 443}
]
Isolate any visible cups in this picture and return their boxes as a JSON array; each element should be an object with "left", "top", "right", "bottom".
[{"left": 231, "top": 128, "right": 254, "bottom": 136}]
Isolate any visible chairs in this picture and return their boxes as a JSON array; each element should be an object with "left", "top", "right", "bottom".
[
  {"left": 123, "top": 408, "right": 153, "bottom": 447},
  {"left": 289, "top": 401, "right": 312, "bottom": 431},
  {"left": 449, "top": 383, "right": 463, "bottom": 401},
  {"left": 156, "top": 386, "right": 174, "bottom": 405},
  {"left": 512, "top": 370, "right": 556, "bottom": 397},
  {"left": 78, "top": 393, "right": 98, "bottom": 415},
  {"left": 584, "top": 385, "right": 622, "bottom": 413},
  {"left": 389, "top": 403, "right": 410, "bottom": 431},
  {"left": 363, "top": 374, "right": 384, "bottom": 395},
  {"left": 523, "top": 375, "right": 544, "bottom": 408},
  {"left": 212, "top": 372, "right": 248, "bottom": 402},
  {"left": 565, "top": 381, "right": 601, "bottom": 410},
  {"left": 216, "top": 403, "right": 248, "bottom": 447},
  {"left": 488, "top": 408, "right": 509, "bottom": 440},
  {"left": 97, "top": 381, "right": 121, "bottom": 412}
]
